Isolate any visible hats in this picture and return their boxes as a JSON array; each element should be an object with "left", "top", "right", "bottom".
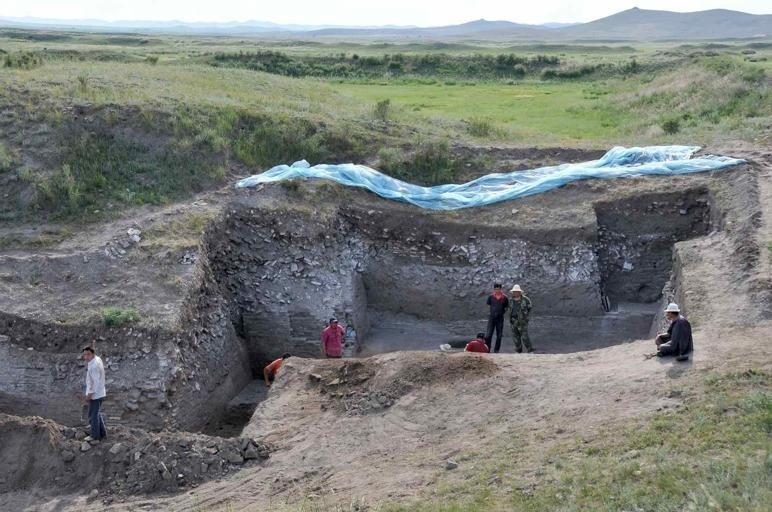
[
  {"left": 509, "top": 285, "right": 523, "bottom": 292},
  {"left": 330, "top": 317, "right": 338, "bottom": 323},
  {"left": 662, "top": 302, "right": 680, "bottom": 313}
]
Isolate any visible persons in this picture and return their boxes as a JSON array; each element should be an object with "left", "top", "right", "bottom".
[
  {"left": 263, "top": 352, "right": 292, "bottom": 390},
  {"left": 485, "top": 281, "right": 509, "bottom": 352},
  {"left": 654, "top": 303, "right": 695, "bottom": 358},
  {"left": 81, "top": 346, "right": 107, "bottom": 442},
  {"left": 508, "top": 284, "right": 537, "bottom": 354},
  {"left": 463, "top": 331, "right": 489, "bottom": 354},
  {"left": 320, "top": 316, "right": 347, "bottom": 358}
]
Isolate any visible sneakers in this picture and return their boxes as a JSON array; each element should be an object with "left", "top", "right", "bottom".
[{"left": 84, "top": 435, "right": 94, "bottom": 441}]
